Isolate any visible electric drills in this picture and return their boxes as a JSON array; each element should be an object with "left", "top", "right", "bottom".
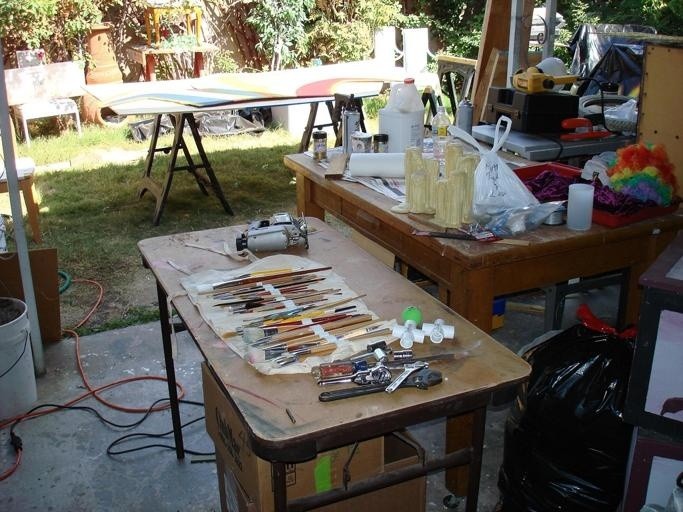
[
  {"left": 512, "top": 67, "right": 580, "bottom": 95},
  {"left": 236, "top": 212, "right": 309, "bottom": 253}
]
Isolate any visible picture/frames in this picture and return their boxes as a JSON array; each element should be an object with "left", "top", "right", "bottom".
[
  {"left": 283, "top": 137, "right": 683, "bottom": 503},
  {"left": 471, "top": 125, "right": 636, "bottom": 166},
  {"left": 79, "top": 59, "right": 441, "bottom": 225},
  {"left": 136, "top": 216, "right": 530, "bottom": 510}
]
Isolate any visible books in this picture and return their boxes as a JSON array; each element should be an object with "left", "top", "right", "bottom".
[
  {"left": 215, "top": 429, "right": 428, "bottom": 511},
  {"left": 198, "top": 362, "right": 388, "bottom": 511},
  {"left": 485, "top": 87, "right": 579, "bottom": 133}
]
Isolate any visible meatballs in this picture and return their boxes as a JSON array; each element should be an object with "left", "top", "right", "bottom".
[{"left": 311, "top": 354, "right": 456, "bottom": 378}]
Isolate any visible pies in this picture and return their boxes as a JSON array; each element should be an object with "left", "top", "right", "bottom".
[{"left": 197, "top": 267, "right": 391, "bottom": 369}]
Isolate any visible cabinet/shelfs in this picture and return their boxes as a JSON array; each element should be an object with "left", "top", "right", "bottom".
[
  {"left": 431, "top": 107, "right": 453, "bottom": 179},
  {"left": 374, "top": 134, "right": 388, "bottom": 153},
  {"left": 312, "top": 131, "right": 326, "bottom": 162}
]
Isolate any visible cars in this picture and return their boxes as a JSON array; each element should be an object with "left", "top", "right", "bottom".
[
  {"left": 350, "top": 134, "right": 372, "bottom": 153},
  {"left": 566, "top": 183, "right": 595, "bottom": 232}
]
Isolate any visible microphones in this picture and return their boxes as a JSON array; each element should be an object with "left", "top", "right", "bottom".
[{"left": 0, "top": 157, "right": 43, "bottom": 243}]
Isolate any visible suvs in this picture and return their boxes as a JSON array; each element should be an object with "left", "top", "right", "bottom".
[
  {"left": 0, "top": 296, "right": 37, "bottom": 424},
  {"left": 378, "top": 78, "right": 425, "bottom": 152}
]
